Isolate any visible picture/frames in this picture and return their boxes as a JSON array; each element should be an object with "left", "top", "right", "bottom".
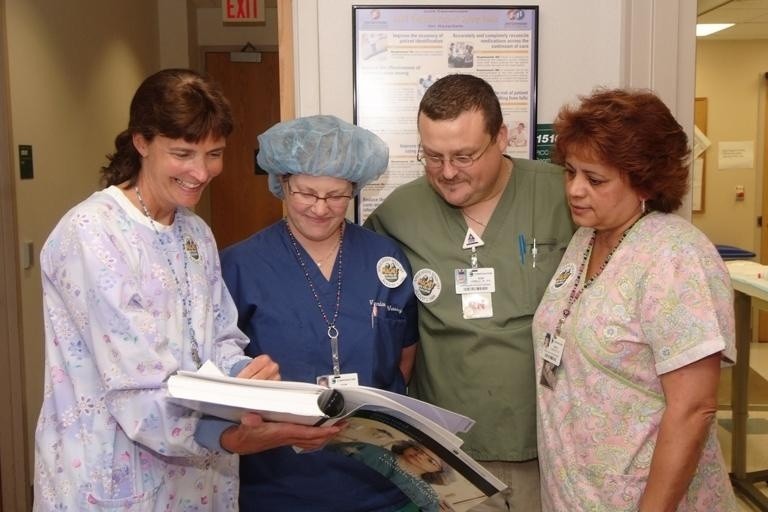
[{"left": 349, "top": 4, "right": 539, "bottom": 229}]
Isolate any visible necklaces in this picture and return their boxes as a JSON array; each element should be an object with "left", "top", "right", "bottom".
[
  {"left": 457, "top": 206, "right": 493, "bottom": 233},
  {"left": 312, "top": 225, "right": 344, "bottom": 269}
]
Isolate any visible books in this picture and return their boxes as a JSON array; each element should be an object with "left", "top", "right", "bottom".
[{"left": 164, "top": 369, "right": 510, "bottom": 512}]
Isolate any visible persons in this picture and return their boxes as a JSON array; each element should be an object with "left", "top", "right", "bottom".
[
  {"left": 361, "top": 75, "right": 587, "bottom": 511},
  {"left": 318, "top": 376, "right": 330, "bottom": 387},
  {"left": 531, "top": 83, "right": 740, "bottom": 510},
  {"left": 333, "top": 433, "right": 455, "bottom": 512},
  {"left": 543, "top": 333, "right": 551, "bottom": 347},
  {"left": 211, "top": 109, "right": 428, "bottom": 511},
  {"left": 32, "top": 67, "right": 351, "bottom": 510}
]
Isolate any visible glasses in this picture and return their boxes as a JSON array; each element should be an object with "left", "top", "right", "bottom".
[
  {"left": 287, "top": 179, "right": 353, "bottom": 209},
  {"left": 416, "top": 134, "right": 495, "bottom": 169}
]
